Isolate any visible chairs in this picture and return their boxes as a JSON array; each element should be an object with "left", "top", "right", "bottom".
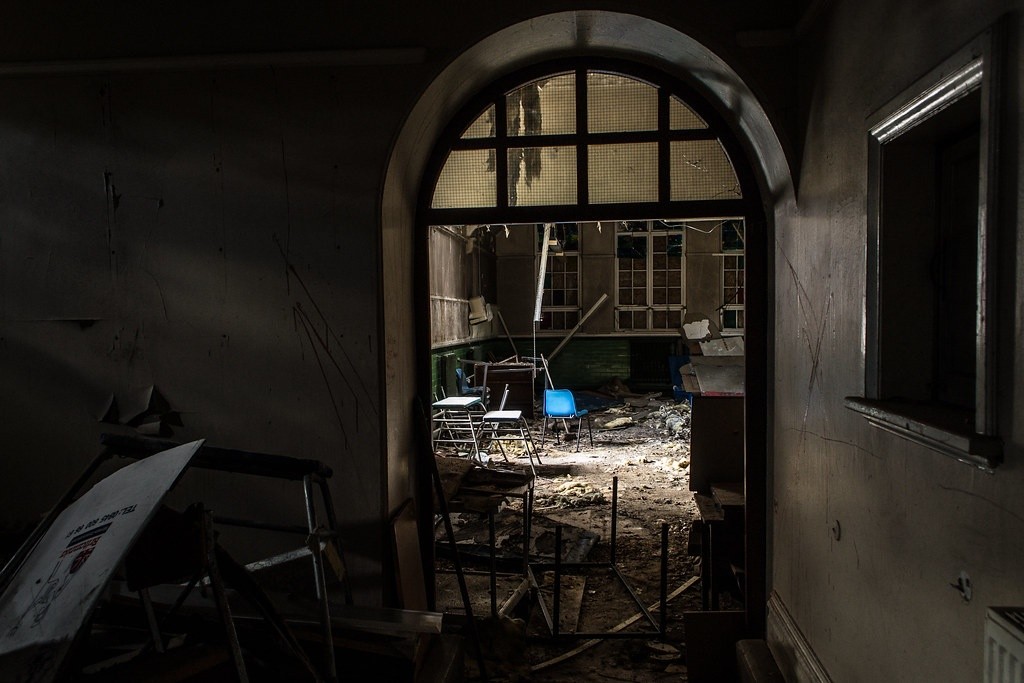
[
  {"left": 541, "top": 389, "right": 594, "bottom": 452},
  {"left": 456, "top": 368, "right": 490, "bottom": 412}
]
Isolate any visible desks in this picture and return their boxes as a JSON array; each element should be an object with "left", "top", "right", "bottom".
[{"left": 432, "top": 383, "right": 542, "bottom": 480}]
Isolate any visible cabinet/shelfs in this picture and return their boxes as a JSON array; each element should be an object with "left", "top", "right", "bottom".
[{"left": 679, "top": 355, "right": 745, "bottom": 494}]
